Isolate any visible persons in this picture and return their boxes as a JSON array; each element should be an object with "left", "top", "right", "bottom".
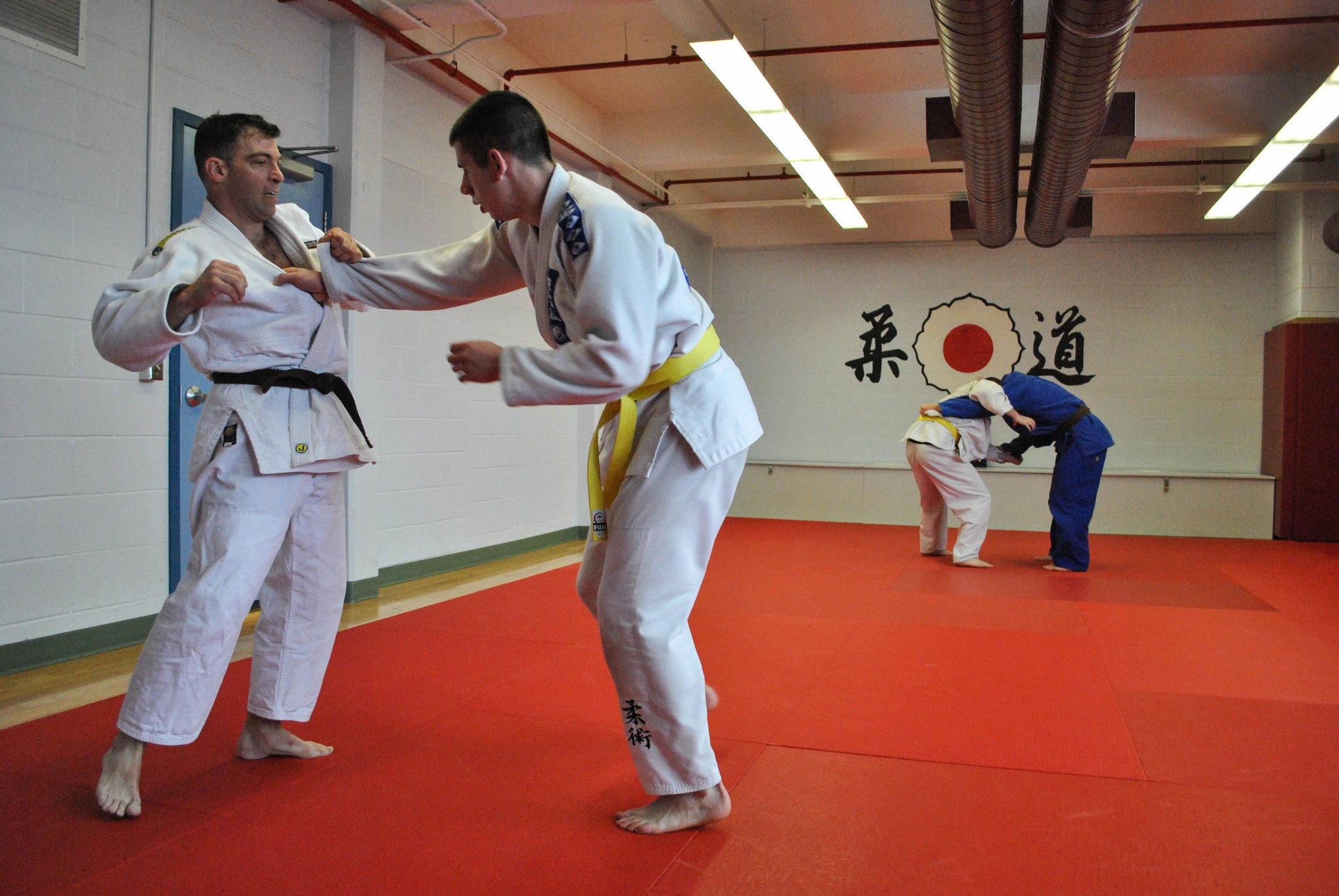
[
  {"left": 273, "top": 89, "right": 764, "bottom": 834},
  {"left": 919, "top": 371, "right": 1115, "bottom": 572},
  {"left": 92, "top": 114, "right": 376, "bottom": 816},
  {"left": 900, "top": 378, "right": 1036, "bottom": 568}
]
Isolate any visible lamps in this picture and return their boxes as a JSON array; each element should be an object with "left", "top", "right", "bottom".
[
  {"left": 654, "top": 0, "right": 869, "bottom": 230},
  {"left": 1204, "top": 66, "right": 1339, "bottom": 220}
]
